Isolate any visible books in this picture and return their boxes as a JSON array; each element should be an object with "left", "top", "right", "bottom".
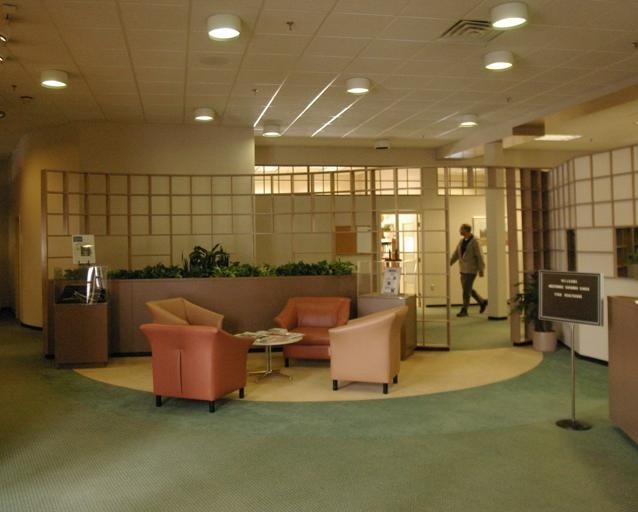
[{"left": 238, "top": 327, "right": 304, "bottom": 343}]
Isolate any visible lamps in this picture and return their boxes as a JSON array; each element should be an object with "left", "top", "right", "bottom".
[
  {"left": 506, "top": 271, "right": 559, "bottom": 352},
  {"left": 491, "top": 3, "right": 528, "bottom": 30},
  {"left": 345, "top": 78, "right": 370, "bottom": 94},
  {"left": 41, "top": 69, "right": 68, "bottom": 89},
  {"left": 484, "top": 50, "right": 513, "bottom": 69},
  {"left": 460, "top": 114, "right": 477, "bottom": 126},
  {"left": 194, "top": 108, "right": 214, "bottom": 120},
  {"left": 262, "top": 125, "right": 281, "bottom": 138},
  {"left": 206, "top": 15, "right": 240, "bottom": 40}
]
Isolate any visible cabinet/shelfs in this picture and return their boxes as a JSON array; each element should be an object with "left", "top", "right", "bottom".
[{"left": 50, "top": 279, "right": 110, "bottom": 367}]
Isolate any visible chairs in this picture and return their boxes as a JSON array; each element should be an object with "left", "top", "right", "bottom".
[
  {"left": 328, "top": 305, "right": 408, "bottom": 394},
  {"left": 140, "top": 323, "right": 255, "bottom": 412},
  {"left": 274, "top": 297, "right": 350, "bottom": 367},
  {"left": 607, "top": 296, "right": 638, "bottom": 445},
  {"left": 146, "top": 298, "right": 223, "bottom": 330}
]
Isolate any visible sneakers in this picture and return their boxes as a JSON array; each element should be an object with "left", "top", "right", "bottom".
[
  {"left": 456, "top": 307, "right": 468, "bottom": 317},
  {"left": 479, "top": 298, "right": 488, "bottom": 314}
]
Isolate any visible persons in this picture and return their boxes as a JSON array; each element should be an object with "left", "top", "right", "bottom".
[{"left": 450, "top": 224, "right": 489, "bottom": 317}]
